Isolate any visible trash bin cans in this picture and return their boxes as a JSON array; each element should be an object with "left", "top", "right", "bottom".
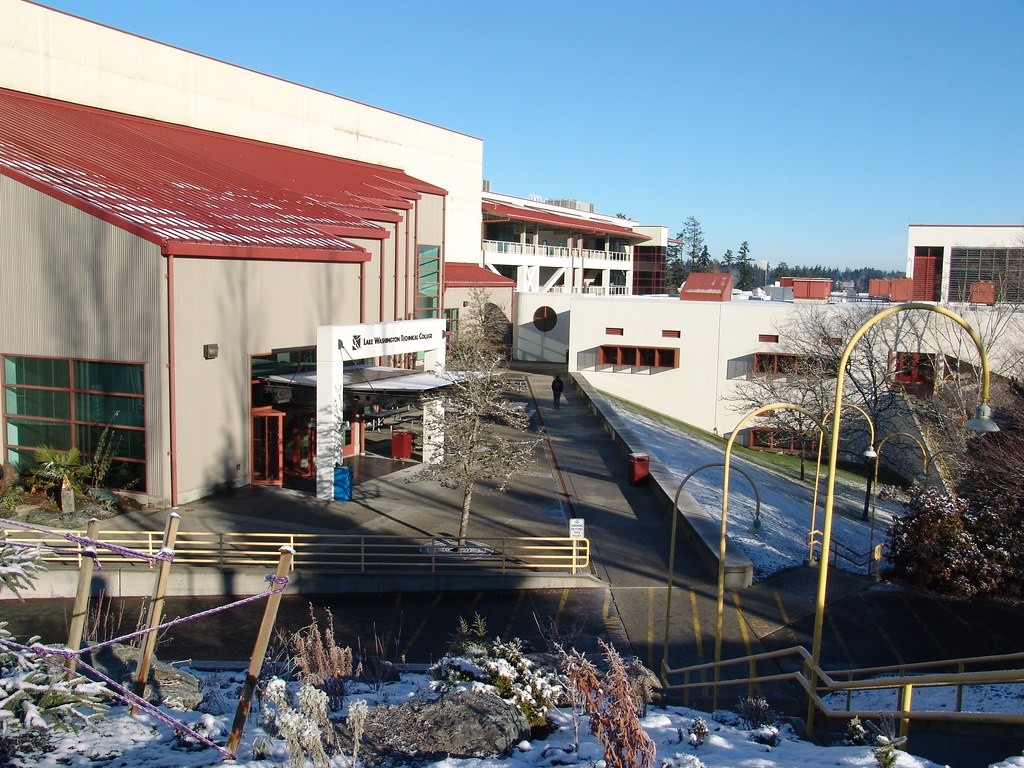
[
  {"left": 390, "top": 429, "right": 413, "bottom": 459},
  {"left": 628, "top": 452, "right": 650, "bottom": 487},
  {"left": 333, "top": 461, "right": 354, "bottom": 501}
]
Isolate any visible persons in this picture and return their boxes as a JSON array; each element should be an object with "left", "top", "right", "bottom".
[{"left": 552, "top": 375, "right": 564, "bottom": 409}]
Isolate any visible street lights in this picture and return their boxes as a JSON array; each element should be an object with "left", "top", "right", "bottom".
[
  {"left": 807, "top": 403, "right": 877, "bottom": 561},
  {"left": 659, "top": 460, "right": 773, "bottom": 691},
  {"left": 710, "top": 402, "right": 841, "bottom": 713},
  {"left": 805, "top": 300, "right": 1004, "bottom": 738},
  {"left": 867, "top": 432, "right": 930, "bottom": 581}
]
